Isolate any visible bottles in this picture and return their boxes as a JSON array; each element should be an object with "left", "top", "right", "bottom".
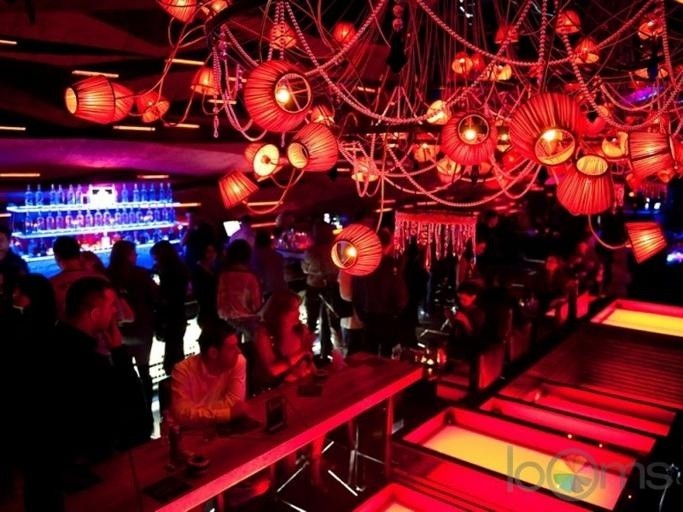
[
  {"left": 28, "top": 226, "right": 175, "bottom": 256},
  {"left": 22, "top": 206, "right": 175, "bottom": 235},
  {"left": 24, "top": 181, "right": 172, "bottom": 207}
]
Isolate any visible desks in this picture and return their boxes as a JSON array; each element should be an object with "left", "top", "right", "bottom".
[{"left": 58, "top": 347, "right": 424, "bottom": 511}]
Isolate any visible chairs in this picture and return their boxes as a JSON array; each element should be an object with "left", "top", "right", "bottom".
[{"left": 155, "top": 376, "right": 175, "bottom": 418}]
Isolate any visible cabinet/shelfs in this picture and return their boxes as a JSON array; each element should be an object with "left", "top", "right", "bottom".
[{"left": 6, "top": 203, "right": 199, "bottom": 262}]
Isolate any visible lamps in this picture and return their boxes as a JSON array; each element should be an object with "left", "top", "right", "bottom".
[{"left": 62, "top": 0, "right": 681, "bottom": 278}]
